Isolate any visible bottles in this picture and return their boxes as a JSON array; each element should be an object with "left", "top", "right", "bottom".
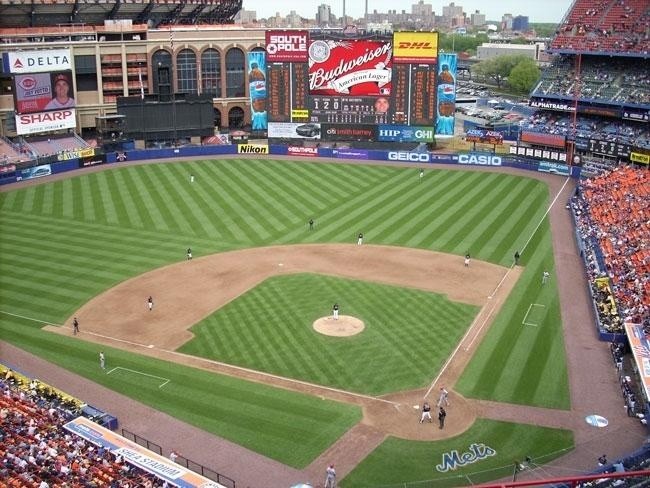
[
  {"left": 438, "top": 64, "right": 455, "bottom": 117},
  {"left": 249, "top": 63, "right": 266, "bottom": 112}
]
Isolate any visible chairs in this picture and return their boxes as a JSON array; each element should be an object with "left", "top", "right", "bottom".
[
  {"left": 0, "top": 366, "right": 175, "bottom": 488},
  {"left": 520, "top": 1, "right": 650, "bottom": 166},
  {"left": 573, "top": 166, "right": 650, "bottom": 334}
]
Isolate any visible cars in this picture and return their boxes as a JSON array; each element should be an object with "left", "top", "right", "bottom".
[
  {"left": 32, "top": 167, "right": 49, "bottom": 176},
  {"left": 456, "top": 63, "right": 535, "bottom": 124},
  {"left": 296, "top": 123, "right": 320, "bottom": 137}
]
